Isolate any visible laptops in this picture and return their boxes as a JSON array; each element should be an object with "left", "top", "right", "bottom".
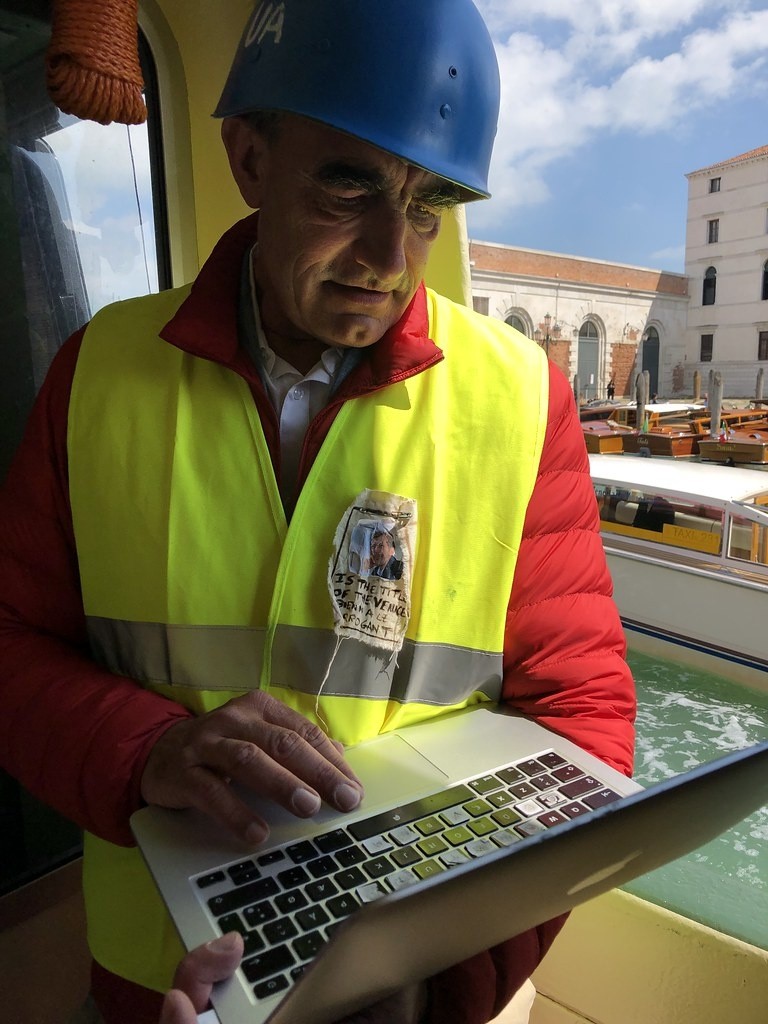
[{"left": 129, "top": 700, "right": 768, "bottom": 1024}]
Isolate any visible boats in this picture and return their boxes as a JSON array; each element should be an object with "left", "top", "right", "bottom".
[
  {"left": 578, "top": 398, "right": 768, "bottom": 468},
  {"left": 570, "top": 452, "right": 767, "bottom": 700}
]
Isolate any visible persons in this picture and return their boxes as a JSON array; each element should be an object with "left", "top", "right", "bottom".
[
  {"left": 1, "top": 0, "right": 634, "bottom": 1024},
  {"left": 703, "top": 392, "right": 708, "bottom": 403},
  {"left": 650, "top": 392, "right": 659, "bottom": 405},
  {"left": 606, "top": 378, "right": 615, "bottom": 401}
]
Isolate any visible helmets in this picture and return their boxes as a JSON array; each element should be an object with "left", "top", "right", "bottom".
[{"left": 215, "top": 1, "right": 500, "bottom": 204}]
